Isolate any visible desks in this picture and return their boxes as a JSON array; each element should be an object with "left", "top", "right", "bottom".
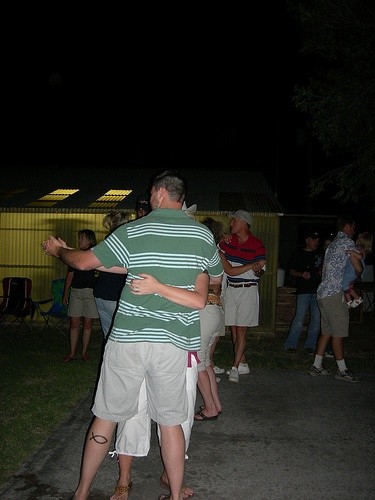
[{"left": 355, "top": 281, "right": 375, "bottom": 313}]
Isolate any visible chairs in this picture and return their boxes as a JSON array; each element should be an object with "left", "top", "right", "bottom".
[
  {"left": 0, "top": 277, "right": 35, "bottom": 337},
  {"left": 38, "top": 279, "right": 69, "bottom": 337}
]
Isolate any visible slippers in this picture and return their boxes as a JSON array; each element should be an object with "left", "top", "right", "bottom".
[
  {"left": 116, "top": 481, "right": 133, "bottom": 498},
  {"left": 63, "top": 356, "right": 77, "bottom": 363},
  {"left": 195, "top": 412, "right": 218, "bottom": 421},
  {"left": 160, "top": 481, "right": 194, "bottom": 500},
  {"left": 81, "top": 355, "right": 90, "bottom": 364},
  {"left": 200, "top": 406, "right": 222, "bottom": 414}
]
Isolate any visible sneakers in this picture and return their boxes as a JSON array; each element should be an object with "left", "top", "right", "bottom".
[
  {"left": 226, "top": 363, "right": 250, "bottom": 375},
  {"left": 310, "top": 365, "right": 331, "bottom": 376},
  {"left": 335, "top": 369, "right": 359, "bottom": 382},
  {"left": 229, "top": 370, "right": 239, "bottom": 384}
]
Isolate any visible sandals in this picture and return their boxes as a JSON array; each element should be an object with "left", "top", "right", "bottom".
[
  {"left": 351, "top": 297, "right": 363, "bottom": 308},
  {"left": 347, "top": 300, "right": 352, "bottom": 307}
]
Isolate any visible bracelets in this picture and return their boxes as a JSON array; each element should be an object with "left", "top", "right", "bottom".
[{"left": 301, "top": 273, "right": 303, "bottom": 276}]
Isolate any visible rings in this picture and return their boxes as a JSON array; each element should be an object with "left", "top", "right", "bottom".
[{"left": 136, "top": 288, "right": 138, "bottom": 291}]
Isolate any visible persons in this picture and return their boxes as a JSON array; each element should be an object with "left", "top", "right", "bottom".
[
  {"left": 63, "top": 230, "right": 98, "bottom": 363},
  {"left": 135, "top": 200, "right": 150, "bottom": 219},
  {"left": 42, "top": 169, "right": 266, "bottom": 500},
  {"left": 92, "top": 208, "right": 130, "bottom": 340},
  {"left": 283, "top": 216, "right": 373, "bottom": 383}
]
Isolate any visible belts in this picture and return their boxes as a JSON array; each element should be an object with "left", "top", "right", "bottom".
[{"left": 228, "top": 282, "right": 257, "bottom": 287}]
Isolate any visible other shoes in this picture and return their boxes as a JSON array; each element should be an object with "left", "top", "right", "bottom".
[
  {"left": 213, "top": 366, "right": 225, "bottom": 373},
  {"left": 305, "top": 347, "right": 313, "bottom": 353},
  {"left": 288, "top": 349, "right": 296, "bottom": 353}
]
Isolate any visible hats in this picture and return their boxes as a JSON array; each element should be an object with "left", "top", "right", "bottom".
[
  {"left": 227, "top": 210, "right": 252, "bottom": 226},
  {"left": 305, "top": 230, "right": 322, "bottom": 239}
]
